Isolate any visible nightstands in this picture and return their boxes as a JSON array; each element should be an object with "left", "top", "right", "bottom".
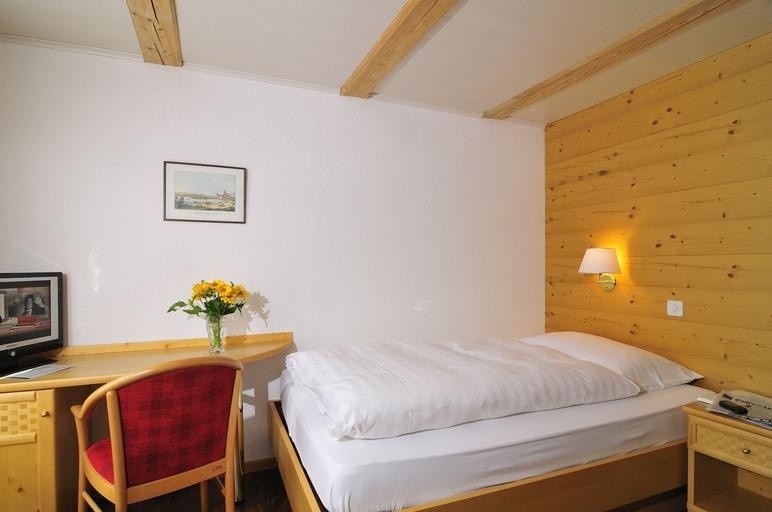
[{"left": 680, "top": 398, "right": 772, "bottom": 512}]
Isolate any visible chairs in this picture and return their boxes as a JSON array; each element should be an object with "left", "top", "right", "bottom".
[{"left": 66, "top": 354, "right": 244, "bottom": 512}]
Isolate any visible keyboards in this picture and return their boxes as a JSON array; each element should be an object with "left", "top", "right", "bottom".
[{"left": 6, "top": 362, "right": 77, "bottom": 380}]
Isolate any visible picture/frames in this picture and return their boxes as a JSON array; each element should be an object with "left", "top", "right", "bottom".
[{"left": 162, "top": 161, "right": 247, "bottom": 222}]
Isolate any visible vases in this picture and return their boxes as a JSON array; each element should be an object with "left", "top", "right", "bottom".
[{"left": 205, "top": 316, "right": 226, "bottom": 353}]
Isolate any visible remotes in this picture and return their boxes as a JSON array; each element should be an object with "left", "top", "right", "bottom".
[{"left": 719, "top": 399, "right": 749, "bottom": 413}]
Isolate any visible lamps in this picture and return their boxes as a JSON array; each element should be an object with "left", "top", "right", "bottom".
[{"left": 578, "top": 248, "right": 622, "bottom": 290}]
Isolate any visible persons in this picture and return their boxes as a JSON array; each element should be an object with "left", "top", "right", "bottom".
[{"left": 9, "top": 292, "right": 49, "bottom": 317}]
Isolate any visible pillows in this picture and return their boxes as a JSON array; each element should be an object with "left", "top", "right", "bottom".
[{"left": 519, "top": 331, "right": 703, "bottom": 392}]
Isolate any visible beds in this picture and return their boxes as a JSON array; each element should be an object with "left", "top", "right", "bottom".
[{"left": 259, "top": 326, "right": 715, "bottom": 510}]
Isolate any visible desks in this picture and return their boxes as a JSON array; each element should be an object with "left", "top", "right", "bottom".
[{"left": 1, "top": 330, "right": 296, "bottom": 512}]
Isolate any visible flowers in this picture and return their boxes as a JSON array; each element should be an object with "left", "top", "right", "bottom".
[{"left": 168, "top": 276, "right": 252, "bottom": 347}]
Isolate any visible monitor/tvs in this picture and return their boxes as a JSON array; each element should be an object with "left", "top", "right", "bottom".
[{"left": 0, "top": 272, "right": 63, "bottom": 378}]
